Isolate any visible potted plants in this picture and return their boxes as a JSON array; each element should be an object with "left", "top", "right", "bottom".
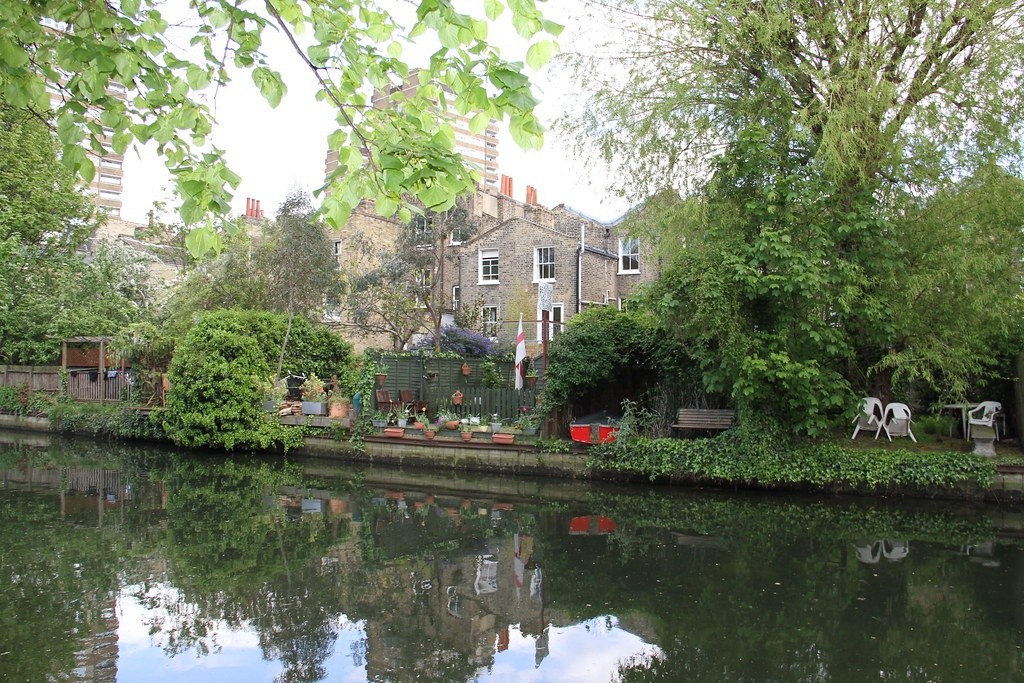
[{"left": 266, "top": 363, "right": 541, "bottom": 441}]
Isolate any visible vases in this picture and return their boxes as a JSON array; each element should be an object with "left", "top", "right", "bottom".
[
  {"left": 384, "top": 428, "right": 405, "bottom": 437},
  {"left": 490, "top": 434, "right": 515, "bottom": 444}
]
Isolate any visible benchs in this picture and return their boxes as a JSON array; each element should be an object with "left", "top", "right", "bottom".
[{"left": 673, "top": 408, "right": 736, "bottom": 438}]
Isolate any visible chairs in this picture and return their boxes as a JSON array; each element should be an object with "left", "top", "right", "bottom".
[{"left": 851, "top": 396, "right": 1010, "bottom": 444}]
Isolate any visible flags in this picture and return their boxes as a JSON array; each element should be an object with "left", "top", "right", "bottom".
[{"left": 515, "top": 314, "right": 526, "bottom": 390}]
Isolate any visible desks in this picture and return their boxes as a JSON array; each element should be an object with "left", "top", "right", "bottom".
[{"left": 944, "top": 402, "right": 982, "bottom": 440}]
